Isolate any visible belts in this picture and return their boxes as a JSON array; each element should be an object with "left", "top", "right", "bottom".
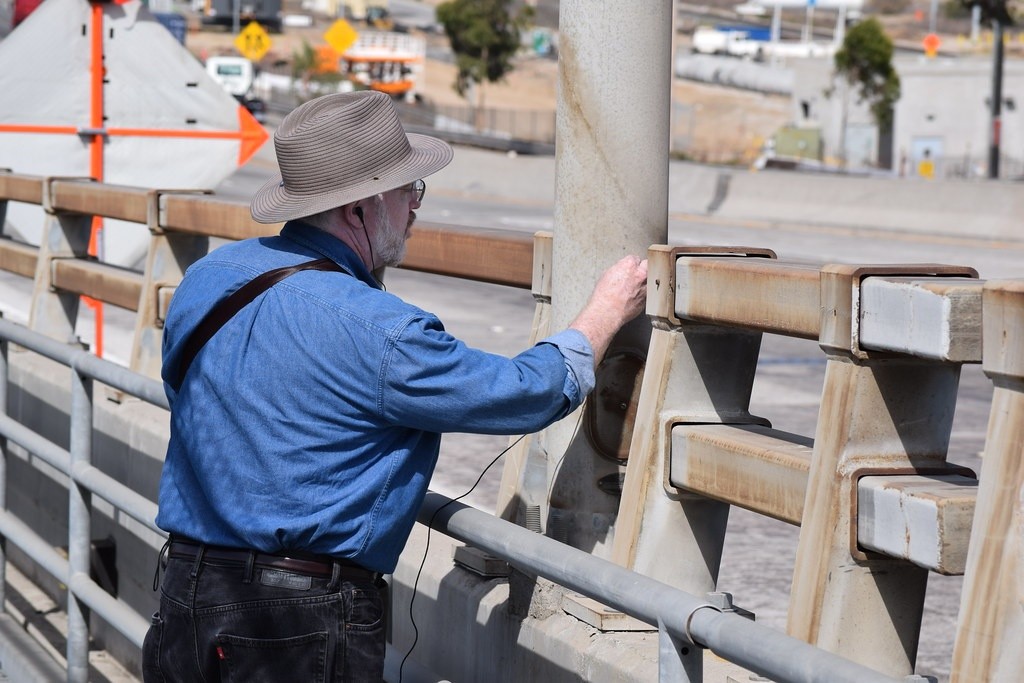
[{"left": 167, "top": 542, "right": 366, "bottom": 581}]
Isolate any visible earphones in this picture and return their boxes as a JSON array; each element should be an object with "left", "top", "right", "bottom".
[{"left": 352, "top": 206, "right": 365, "bottom": 226}]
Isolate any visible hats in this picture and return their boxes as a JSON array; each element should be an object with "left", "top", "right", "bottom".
[{"left": 250, "top": 89, "right": 454, "bottom": 225}]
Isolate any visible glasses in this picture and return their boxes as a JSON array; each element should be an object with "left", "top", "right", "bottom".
[{"left": 395, "top": 179, "right": 426, "bottom": 202}]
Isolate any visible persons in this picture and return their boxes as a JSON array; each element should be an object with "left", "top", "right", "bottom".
[{"left": 141, "top": 90, "right": 647, "bottom": 683}]
